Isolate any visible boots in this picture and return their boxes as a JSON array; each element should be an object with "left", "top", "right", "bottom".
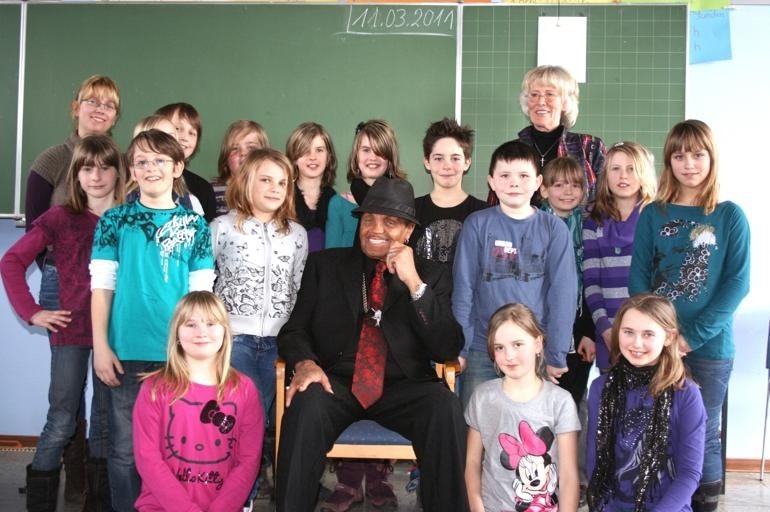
[
  {"left": 25, "top": 437, "right": 112, "bottom": 511},
  {"left": 365, "top": 458, "right": 399, "bottom": 511},
  {"left": 320, "top": 461, "right": 364, "bottom": 512}
]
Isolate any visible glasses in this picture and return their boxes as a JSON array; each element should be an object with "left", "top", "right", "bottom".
[
  {"left": 132, "top": 159, "right": 177, "bottom": 169},
  {"left": 80, "top": 98, "right": 118, "bottom": 113},
  {"left": 526, "top": 91, "right": 559, "bottom": 103}
]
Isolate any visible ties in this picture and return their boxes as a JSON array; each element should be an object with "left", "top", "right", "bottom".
[{"left": 350, "top": 259, "right": 388, "bottom": 410}]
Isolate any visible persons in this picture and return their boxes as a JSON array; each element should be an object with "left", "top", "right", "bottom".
[{"left": 0, "top": 65, "right": 751, "bottom": 512}]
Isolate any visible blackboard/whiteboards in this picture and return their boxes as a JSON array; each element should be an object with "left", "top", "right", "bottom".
[{"left": 0, "top": 2, "right": 690, "bottom": 219}]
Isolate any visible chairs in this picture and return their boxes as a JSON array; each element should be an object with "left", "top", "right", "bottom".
[{"left": 273, "top": 353, "right": 460, "bottom": 511}]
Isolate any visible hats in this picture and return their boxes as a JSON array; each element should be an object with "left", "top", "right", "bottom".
[{"left": 350, "top": 176, "right": 423, "bottom": 227}]
